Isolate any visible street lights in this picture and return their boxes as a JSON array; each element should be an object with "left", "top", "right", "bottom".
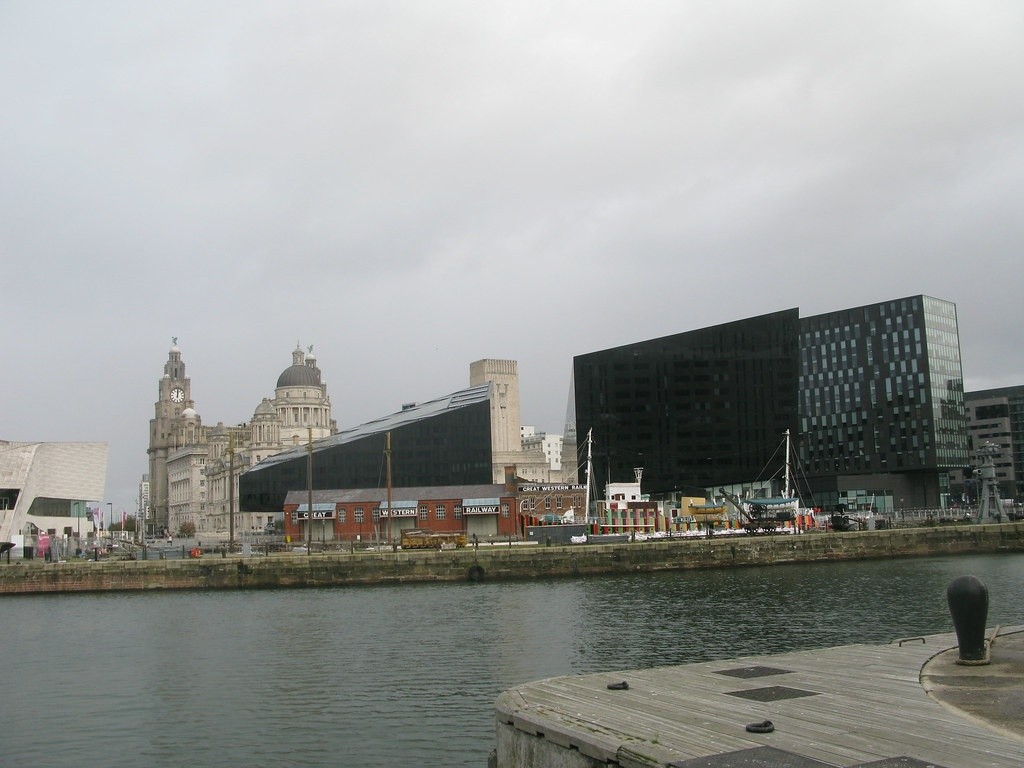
[
  {"left": 73, "top": 502, "right": 80, "bottom": 548},
  {"left": 107, "top": 503, "right": 113, "bottom": 542}
]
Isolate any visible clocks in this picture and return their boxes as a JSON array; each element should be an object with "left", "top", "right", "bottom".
[{"left": 169, "top": 388, "right": 185, "bottom": 403}]
